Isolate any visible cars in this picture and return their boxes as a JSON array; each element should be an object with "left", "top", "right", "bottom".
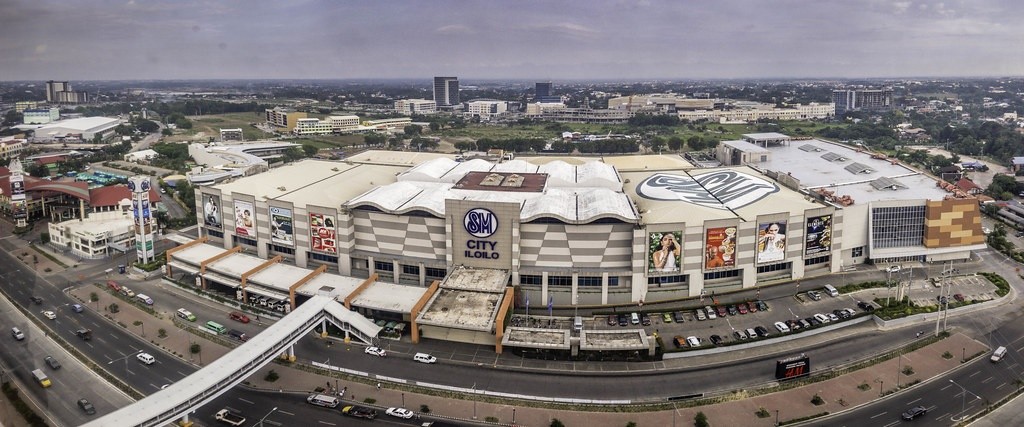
[
  {"left": 673, "top": 312, "right": 684, "bottom": 323},
  {"left": 857, "top": 301, "right": 874, "bottom": 311},
  {"left": 942, "top": 268, "right": 959, "bottom": 276},
  {"left": 710, "top": 334, "right": 722, "bottom": 344},
  {"left": 385, "top": 406, "right": 413, "bottom": 420},
  {"left": 736, "top": 304, "right": 747, "bottom": 314},
  {"left": 825, "top": 313, "right": 839, "bottom": 321},
  {"left": 696, "top": 310, "right": 706, "bottom": 321},
  {"left": 726, "top": 305, "right": 736, "bottom": 315},
  {"left": 833, "top": 310, "right": 850, "bottom": 319},
  {"left": 756, "top": 300, "right": 767, "bottom": 311},
  {"left": 843, "top": 307, "right": 856, "bottom": 317},
  {"left": 607, "top": 315, "right": 616, "bottom": 326},
  {"left": 44, "top": 310, "right": 56, "bottom": 319},
  {"left": 414, "top": 353, "right": 437, "bottom": 364},
  {"left": 687, "top": 336, "right": 700, "bottom": 347},
  {"left": 796, "top": 319, "right": 810, "bottom": 328},
  {"left": 663, "top": 314, "right": 672, "bottom": 323},
  {"left": 755, "top": 326, "right": 770, "bottom": 337},
  {"left": 885, "top": 265, "right": 900, "bottom": 273},
  {"left": 733, "top": 329, "right": 747, "bottom": 340},
  {"left": 745, "top": 302, "right": 757, "bottom": 312},
  {"left": 808, "top": 290, "right": 821, "bottom": 301},
  {"left": 953, "top": 293, "right": 964, "bottom": 301},
  {"left": 43, "top": 355, "right": 61, "bottom": 369},
  {"left": 617, "top": 314, "right": 628, "bottom": 326},
  {"left": 136, "top": 352, "right": 155, "bottom": 365},
  {"left": 716, "top": 306, "right": 726, "bottom": 317},
  {"left": 705, "top": 306, "right": 716, "bottom": 319},
  {"left": 365, "top": 346, "right": 386, "bottom": 357},
  {"left": 674, "top": 335, "right": 686, "bottom": 348},
  {"left": 775, "top": 322, "right": 791, "bottom": 333},
  {"left": 106, "top": 280, "right": 121, "bottom": 292},
  {"left": 77, "top": 398, "right": 97, "bottom": 415},
  {"left": 229, "top": 311, "right": 249, "bottom": 323},
  {"left": 785, "top": 320, "right": 800, "bottom": 330},
  {"left": 72, "top": 304, "right": 83, "bottom": 313},
  {"left": 937, "top": 295, "right": 949, "bottom": 304},
  {"left": 804, "top": 318, "right": 818, "bottom": 325}
]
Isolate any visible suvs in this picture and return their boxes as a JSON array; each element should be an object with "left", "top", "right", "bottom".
[{"left": 932, "top": 277, "right": 941, "bottom": 288}]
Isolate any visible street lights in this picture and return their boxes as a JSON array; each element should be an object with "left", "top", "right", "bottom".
[{"left": 251, "top": 407, "right": 278, "bottom": 427}]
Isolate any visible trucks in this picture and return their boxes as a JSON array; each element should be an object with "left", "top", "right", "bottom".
[
  {"left": 77, "top": 330, "right": 91, "bottom": 340},
  {"left": 215, "top": 409, "right": 246, "bottom": 427},
  {"left": 31, "top": 368, "right": 52, "bottom": 388},
  {"left": 342, "top": 406, "right": 374, "bottom": 418},
  {"left": 307, "top": 394, "right": 339, "bottom": 408}
]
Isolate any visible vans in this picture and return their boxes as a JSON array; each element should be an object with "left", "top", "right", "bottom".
[
  {"left": 745, "top": 329, "right": 757, "bottom": 338},
  {"left": 137, "top": 293, "right": 154, "bottom": 306},
  {"left": 206, "top": 322, "right": 227, "bottom": 334},
  {"left": 573, "top": 316, "right": 583, "bottom": 331},
  {"left": 630, "top": 312, "right": 640, "bottom": 324},
  {"left": 824, "top": 284, "right": 839, "bottom": 297},
  {"left": 640, "top": 312, "right": 651, "bottom": 325},
  {"left": 177, "top": 308, "right": 196, "bottom": 321},
  {"left": 11, "top": 326, "right": 25, "bottom": 340},
  {"left": 990, "top": 346, "right": 1007, "bottom": 363},
  {"left": 120, "top": 287, "right": 135, "bottom": 298},
  {"left": 813, "top": 313, "right": 829, "bottom": 323},
  {"left": 228, "top": 329, "right": 245, "bottom": 339}
]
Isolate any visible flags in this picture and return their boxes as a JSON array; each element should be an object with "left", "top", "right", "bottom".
[
  {"left": 526, "top": 295, "right": 529, "bottom": 305},
  {"left": 548, "top": 295, "right": 552, "bottom": 313}
]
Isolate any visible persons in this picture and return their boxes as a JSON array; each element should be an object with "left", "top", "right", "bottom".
[
  {"left": 639, "top": 300, "right": 643, "bottom": 312},
  {"left": 711, "top": 290, "right": 714, "bottom": 298},
  {"left": 271, "top": 215, "right": 284, "bottom": 240},
  {"left": 242, "top": 210, "right": 252, "bottom": 228},
  {"left": 701, "top": 292, "right": 705, "bottom": 303},
  {"left": 204, "top": 196, "right": 218, "bottom": 223},
  {"left": 652, "top": 233, "right": 681, "bottom": 272},
  {"left": 378, "top": 383, "right": 381, "bottom": 390},
  {"left": 326, "top": 381, "right": 347, "bottom": 397},
  {"left": 706, "top": 246, "right": 724, "bottom": 268},
  {"left": 759, "top": 222, "right": 785, "bottom": 261}
]
